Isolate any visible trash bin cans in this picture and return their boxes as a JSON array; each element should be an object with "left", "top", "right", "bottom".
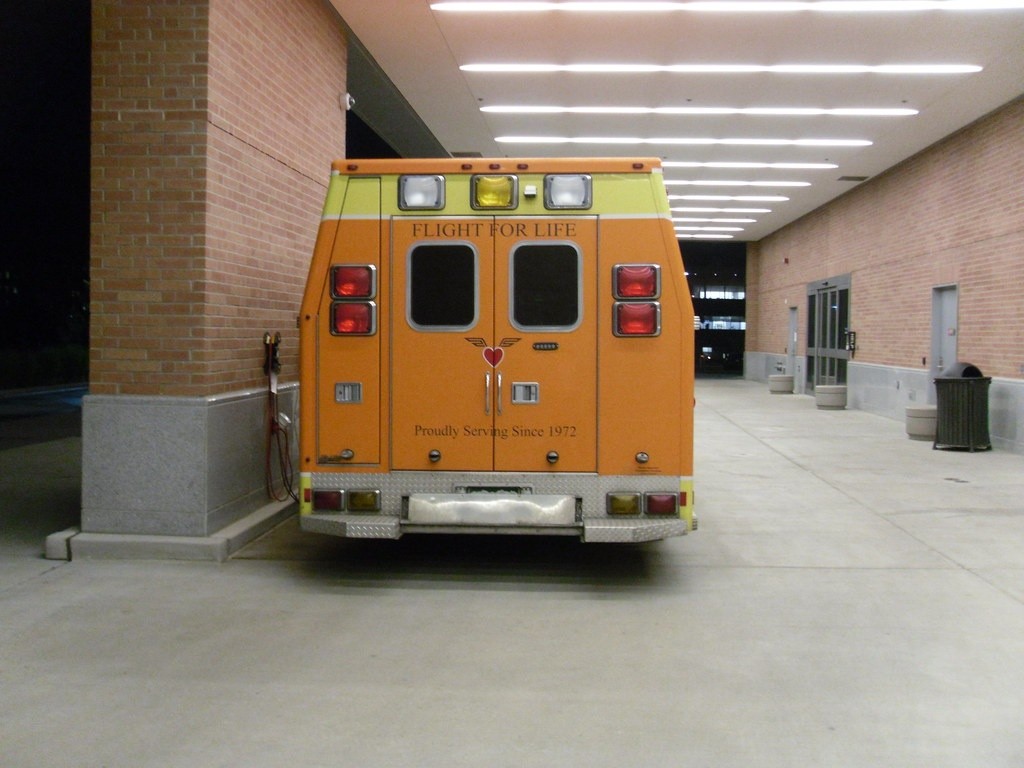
[{"left": 932, "top": 361, "right": 993, "bottom": 453}]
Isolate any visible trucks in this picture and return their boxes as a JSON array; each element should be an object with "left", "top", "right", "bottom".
[{"left": 298, "top": 150, "right": 698, "bottom": 542}]
